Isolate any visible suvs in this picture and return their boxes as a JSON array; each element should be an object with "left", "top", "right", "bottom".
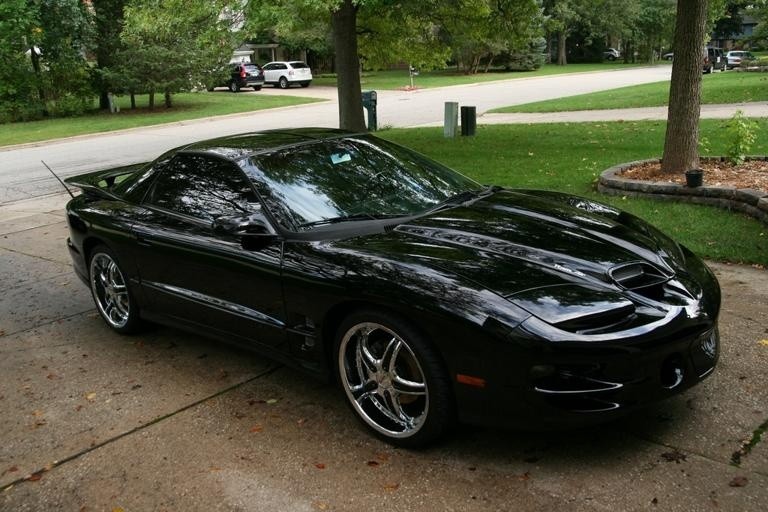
[
  {"left": 206, "top": 62, "right": 264, "bottom": 91},
  {"left": 260, "top": 61, "right": 312, "bottom": 88}
]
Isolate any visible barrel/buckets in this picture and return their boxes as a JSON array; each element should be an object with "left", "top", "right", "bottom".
[{"left": 685, "top": 169, "right": 703, "bottom": 188}]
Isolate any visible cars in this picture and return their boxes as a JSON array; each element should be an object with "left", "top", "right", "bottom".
[
  {"left": 663, "top": 53, "right": 674, "bottom": 61},
  {"left": 66, "top": 126, "right": 721, "bottom": 451},
  {"left": 603, "top": 47, "right": 620, "bottom": 61},
  {"left": 703, "top": 47, "right": 755, "bottom": 74}
]
